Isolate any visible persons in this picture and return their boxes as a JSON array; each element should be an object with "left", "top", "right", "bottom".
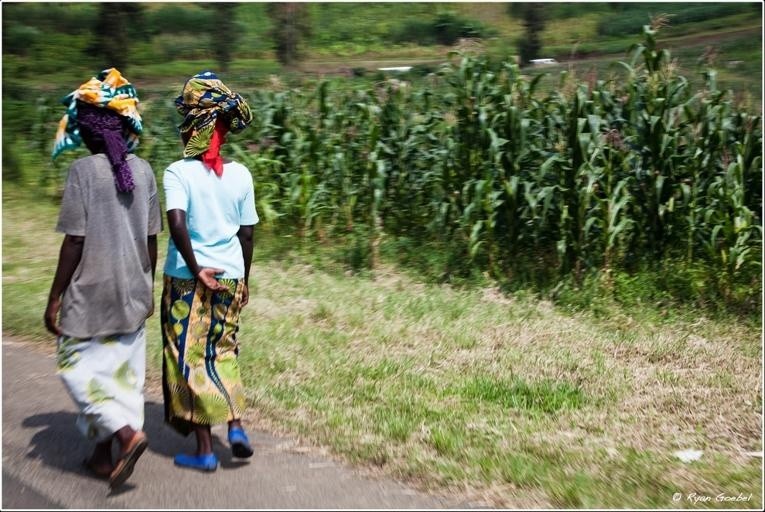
[
  {"left": 157, "top": 71, "right": 259, "bottom": 471},
  {"left": 41, "top": 66, "right": 164, "bottom": 488}
]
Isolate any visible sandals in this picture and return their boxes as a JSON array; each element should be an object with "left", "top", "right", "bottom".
[
  {"left": 228, "top": 428, "right": 254, "bottom": 458},
  {"left": 175, "top": 449, "right": 217, "bottom": 471}
]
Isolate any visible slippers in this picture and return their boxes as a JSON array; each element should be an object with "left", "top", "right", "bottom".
[
  {"left": 81, "top": 457, "right": 114, "bottom": 477},
  {"left": 109, "top": 431, "right": 149, "bottom": 488}
]
